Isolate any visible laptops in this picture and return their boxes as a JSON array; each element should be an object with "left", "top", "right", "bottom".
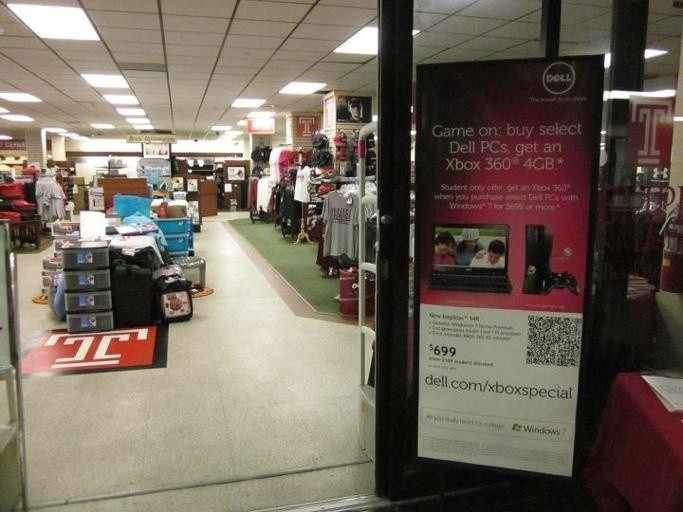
[{"left": 429, "top": 222, "right": 513, "bottom": 293}]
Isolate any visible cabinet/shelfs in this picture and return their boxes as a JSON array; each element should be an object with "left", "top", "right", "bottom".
[
  {"left": 153, "top": 180, "right": 218, "bottom": 217},
  {"left": 0, "top": 181, "right": 41, "bottom": 250},
  {"left": 97, "top": 177, "right": 148, "bottom": 210}
]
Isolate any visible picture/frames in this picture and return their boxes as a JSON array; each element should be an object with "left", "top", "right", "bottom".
[
  {"left": 187, "top": 179, "right": 198, "bottom": 192},
  {"left": 142, "top": 142, "right": 171, "bottom": 159},
  {"left": 173, "top": 177, "right": 183, "bottom": 188},
  {"left": 228, "top": 167, "right": 246, "bottom": 180}
]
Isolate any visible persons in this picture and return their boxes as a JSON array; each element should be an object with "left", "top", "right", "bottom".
[
  {"left": 238, "top": 170, "right": 243, "bottom": 178},
  {"left": 433, "top": 228, "right": 505, "bottom": 268},
  {"left": 348, "top": 98, "right": 364, "bottom": 123},
  {"left": 172, "top": 180, "right": 179, "bottom": 188},
  {"left": 47, "top": 155, "right": 60, "bottom": 175}
]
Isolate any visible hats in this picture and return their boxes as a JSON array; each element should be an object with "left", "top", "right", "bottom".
[
  {"left": 334, "top": 132, "right": 348, "bottom": 147},
  {"left": 336, "top": 151, "right": 347, "bottom": 161},
  {"left": 312, "top": 133, "right": 326, "bottom": 147},
  {"left": 462, "top": 228, "right": 480, "bottom": 241},
  {"left": 309, "top": 151, "right": 332, "bottom": 167},
  {"left": 352, "top": 133, "right": 376, "bottom": 171},
  {"left": 348, "top": 97, "right": 362, "bottom": 106}
]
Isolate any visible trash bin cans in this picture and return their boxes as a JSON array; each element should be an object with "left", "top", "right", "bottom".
[
  {"left": 337, "top": 263, "right": 378, "bottom": 320},
  {"left": 230, "top": 199, "right": 237, "bottom": 212}
]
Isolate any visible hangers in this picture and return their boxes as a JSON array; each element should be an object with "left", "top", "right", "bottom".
[{"left": 324, "top": 182, "right": 378, "bottom": 202}]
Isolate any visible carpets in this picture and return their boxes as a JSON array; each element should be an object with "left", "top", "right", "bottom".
[
  {"left": 32, "top": 285, "right": 214, "bottom": 304},
  {"left": 12, "top": 321, "right": 169, "bottom": 379}
]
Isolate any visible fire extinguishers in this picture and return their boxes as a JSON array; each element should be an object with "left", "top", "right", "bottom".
[{"left": 659, "top": 185, "right": 683, "bottom": 293}]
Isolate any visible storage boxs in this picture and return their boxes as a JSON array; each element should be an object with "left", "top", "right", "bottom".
[{"left": 41, "top": 195, "right": 206, "bottom": 333}]
